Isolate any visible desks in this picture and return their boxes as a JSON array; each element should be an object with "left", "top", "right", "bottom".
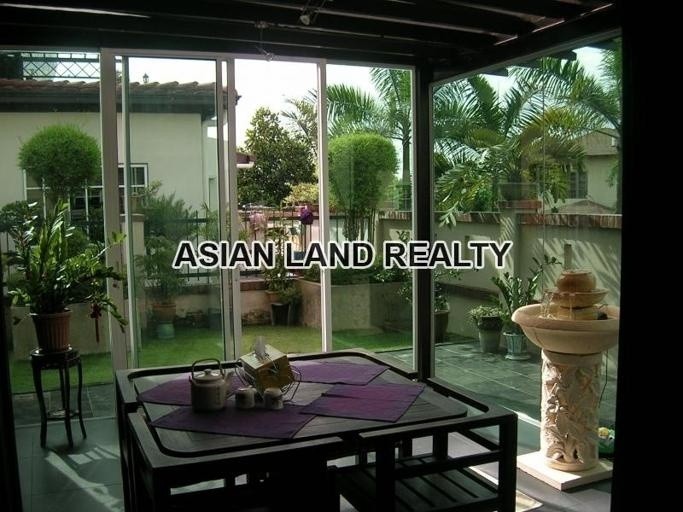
[{"left": 113, "top": 347, "right": 517, "bottom": 510}]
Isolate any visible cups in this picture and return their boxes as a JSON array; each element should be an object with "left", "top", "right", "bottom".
[
  {"left": 263, "top": 387, "right": 285, "bottom": 411},
  {"left": 234, "top": 386, "right": 257, "bottom": 410}
]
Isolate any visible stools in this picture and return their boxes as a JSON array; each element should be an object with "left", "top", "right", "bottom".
[{"left": 29, "top": 348, "right": 89, "bottom": 449}]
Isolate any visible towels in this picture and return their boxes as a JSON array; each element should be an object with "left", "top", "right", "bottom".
[
  {"left": 135, "top": 364, "right": 249, "bottom": 406},
  {"left": 149, "top": 400, "right": 317, "bottom": 441},
  {"left": 298, "top": 383, "right": 426, "bottom": 422},
  {"left": 295, "top": 356, "right": 392, "bottom": 387}
]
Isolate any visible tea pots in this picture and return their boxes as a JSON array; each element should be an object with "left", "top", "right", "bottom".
[{"left": 188, "top": 357, "right": 235, "bottom": 413}]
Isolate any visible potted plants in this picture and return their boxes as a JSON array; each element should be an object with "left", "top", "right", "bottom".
[
  {"left": 134, "top": 177, "right": 201, "bottom": 340},
  {"left": 375, "top": 231, "right": 451, "bottom": 342},
  {"left": 0, "top": 201, "right": 127, "bottom": 353},
  {"left": 470, "top": 302, "right": 502, "bottom": 353},
  {"left": 489, "top": 253, "right": 562, "bottom": 360},
  {"left": 267, "top": 225, "right": 302, "bottom": 326}
]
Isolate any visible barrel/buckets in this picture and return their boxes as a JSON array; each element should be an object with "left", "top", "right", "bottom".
[{"left": 26, "top": 307, "right": 71, "bottom": 354}]
